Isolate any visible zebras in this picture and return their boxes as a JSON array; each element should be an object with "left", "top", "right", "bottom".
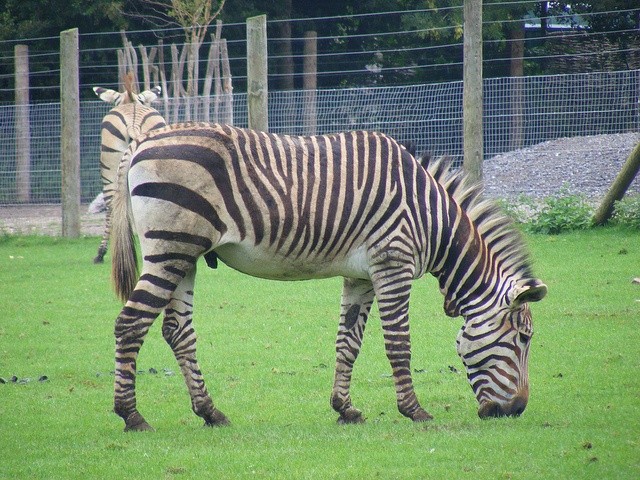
[
  {"left": 109, "top": 120, "right": 550, "bottom": 436},
  {"left": 90, "top": 73, "right": 169, "bottom": 265}
]
